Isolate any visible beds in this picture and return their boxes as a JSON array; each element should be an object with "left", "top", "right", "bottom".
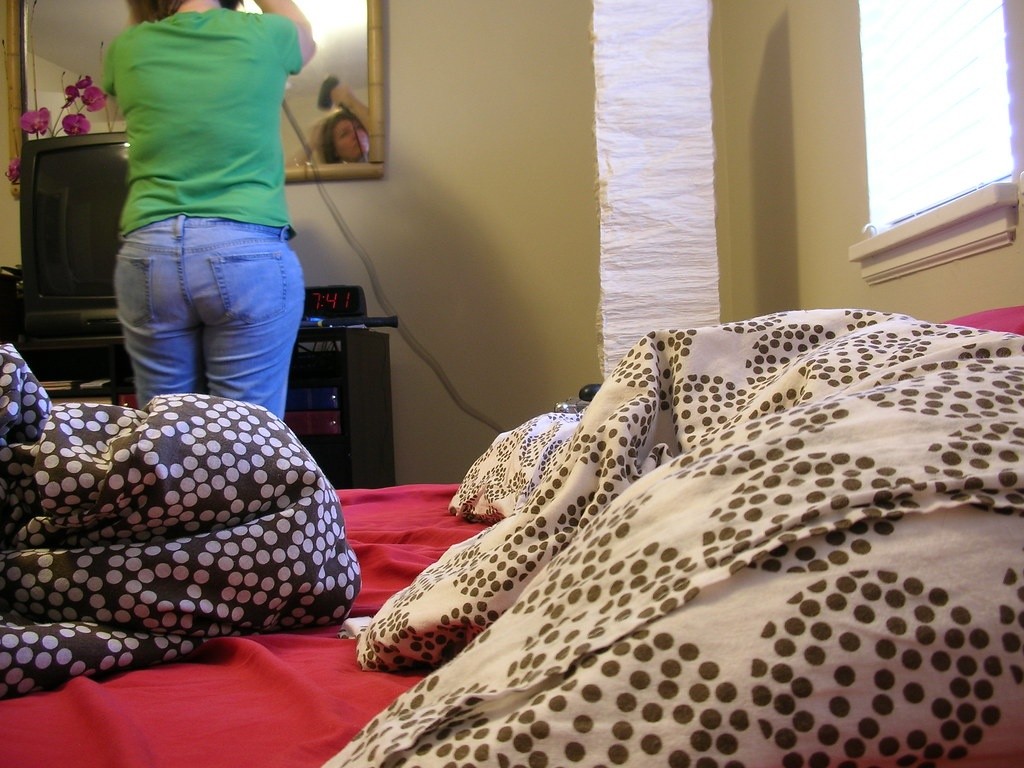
[{"left": 0, "top": 304, "right": 1024, "bottom": 767}]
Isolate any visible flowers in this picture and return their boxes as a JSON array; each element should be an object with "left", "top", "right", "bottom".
[{"left": 3, "top": 75, "right": 107, "bottom": 184}]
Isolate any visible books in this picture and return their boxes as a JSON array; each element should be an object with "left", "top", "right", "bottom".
[{"left": 37, "top": 379, "right": 88, "bottom": 391}]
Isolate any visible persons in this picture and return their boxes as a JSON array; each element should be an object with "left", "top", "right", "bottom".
[
  {"left": 99, "top": 0, "right": 316, "bottom": 421},
  {"left": 280, "top": 75, "right": 374, "bottom": 166}
]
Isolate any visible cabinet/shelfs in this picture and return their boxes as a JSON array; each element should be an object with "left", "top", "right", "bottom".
[{"left": 6, "top": 323, "right": 393, "bottom": 491}]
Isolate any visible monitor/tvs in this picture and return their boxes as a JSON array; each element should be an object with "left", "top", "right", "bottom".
[{"left": 20, "top": 131, "right": 129, "bottom": 337}]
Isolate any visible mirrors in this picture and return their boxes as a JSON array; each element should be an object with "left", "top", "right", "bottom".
[{"left": 5, "top": 0, "right": 387, "bottom": 201}]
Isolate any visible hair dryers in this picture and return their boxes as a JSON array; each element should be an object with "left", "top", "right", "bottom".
[{"left": 316, "top": 72, "right": 346, "bottom": 114}]
